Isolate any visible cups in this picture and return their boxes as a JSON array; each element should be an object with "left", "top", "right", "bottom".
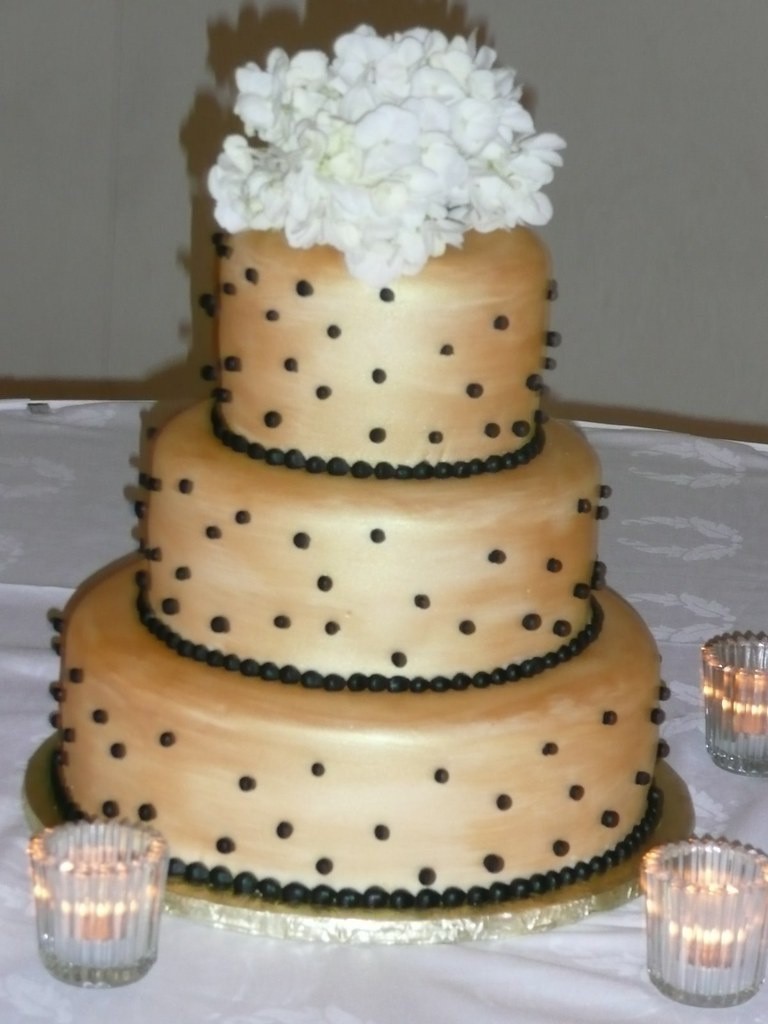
[
  {"left": 638, "top": 834, "right": 768, "bottom": 1007},
  {"left": 30, "top": 819, "right": 170, "bottom": 990},
  {"left": 702, "top": 631, "right": 768, "bottom": 777}
]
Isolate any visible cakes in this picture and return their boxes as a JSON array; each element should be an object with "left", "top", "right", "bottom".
[{"left": 54, "top": 226, "right": 670, "bottom": 906}]
